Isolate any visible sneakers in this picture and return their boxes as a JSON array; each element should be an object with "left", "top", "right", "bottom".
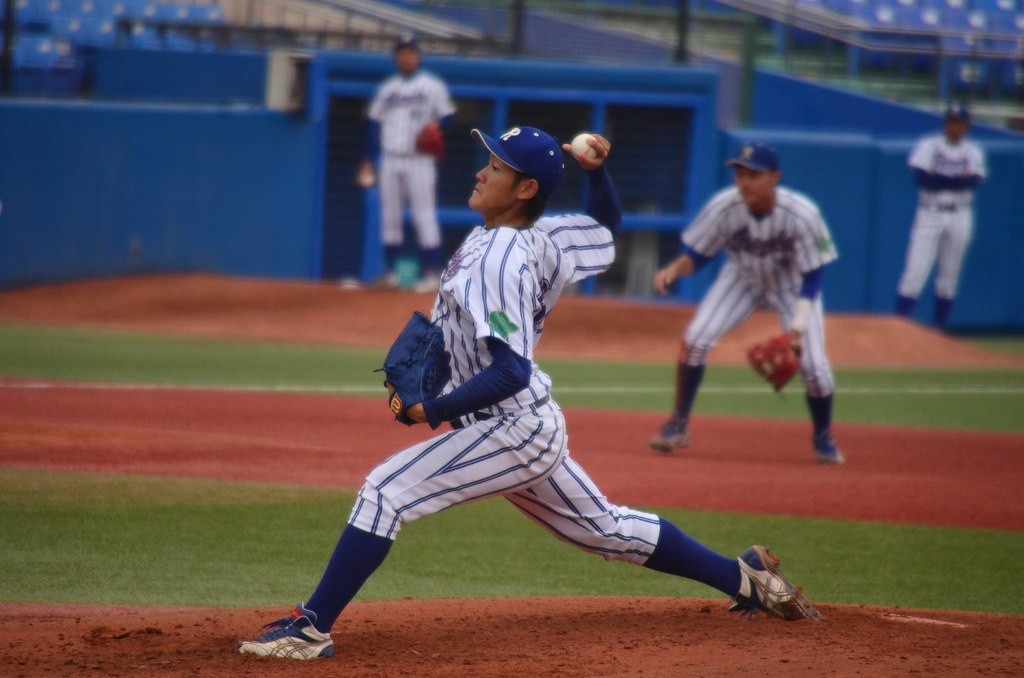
[
  {"left": 731, "top": 545, "right": 824, "bottom": 622},
  {"left": 813, "top": 433, "right": 843, "bottom": 463},
  {"left": 235, "top": 602, "right": 333, "bottom": 660},
  {"left": 650, "top": 421, "right": 690, "bottom": 450}
]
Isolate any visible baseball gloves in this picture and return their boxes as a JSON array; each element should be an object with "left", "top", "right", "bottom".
[
  {"left": 747, "top": 331, "right": 802, "bottom": 389},
  {"left": 417, "top": 125, "right": 447, "bottom": 157},
  {"left": 380, "top": 310, "right": 453, "bottom": 426}
]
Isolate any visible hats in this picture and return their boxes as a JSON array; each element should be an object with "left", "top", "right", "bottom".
[
  {"left": 945, "top": 104, "right": 969, "bottom": 123},
  {"left": 727, "top": 143, "right": 778, "bottom": 173},
  {"left": 471, "top": 126, "right": 565, "bottom": 196},
  {"left": 394, "top": 34, "right": 419, "bottom": 51}
]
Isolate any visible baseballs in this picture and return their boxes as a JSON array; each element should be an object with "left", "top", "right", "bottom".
[{"left": 571, "top": 133, "right": 597, "bottom": 163}]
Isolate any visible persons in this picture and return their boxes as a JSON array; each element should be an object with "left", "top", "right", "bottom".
[
  {"left": 890, "top": 104, "right": 986, "bottom": 332},
  {"left": 649, "top": 143, "right": 844, "bottom": 465},
  {"left": 356, "top": 31, "right": 456, "bottom": 293},
  {"left": 235, "top": 127, "right": 822, "bottom": 660}
]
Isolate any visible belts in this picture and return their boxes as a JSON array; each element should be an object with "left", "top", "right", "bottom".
[{"left": 450, "top": 411, "right": 491, "bottom": 430}]
